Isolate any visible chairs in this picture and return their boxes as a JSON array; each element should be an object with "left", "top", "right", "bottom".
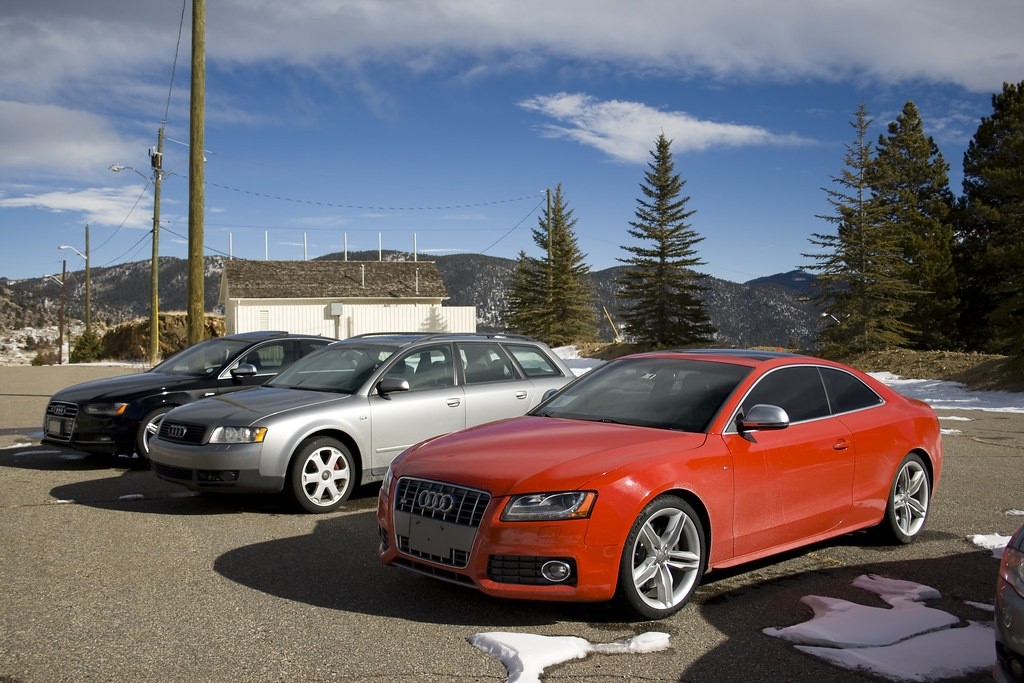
[
  {"left": 483, "top": 360, "right": 513, "bottom": 381},
  {"left": 745, "top": 377, "right": 800, "bottom": 421},
  {"left": 246, "top": 352, "right": 263, "bottom": 371},
  {"left": 657, "top": 372, "right": 709, "bottom": 412},
  {"left": 388, "top": 359, "right": 412, "bottom": 382},
  {"left": 414, "top": 361, "right": 447, "bottom": 389}
]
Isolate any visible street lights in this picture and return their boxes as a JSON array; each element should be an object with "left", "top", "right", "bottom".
[
  {"left": 44, "top": 274, "right": 65, "bottom": 364},
  {"left": 58, "top": 244, "right": 92, "bottom": 336},
  {"left": 108, "top": 164, "right": 159, "bottom": 368}
]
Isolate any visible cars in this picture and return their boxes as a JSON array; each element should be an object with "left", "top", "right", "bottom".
[
  {"left": 41, "top": 331, "right": 366, "bottom": 462},
  {"left": 147, "top": 333, "right": 579, "bottom": 513},
  {"left": 377, "top": 349, "right": 941, "bottom": 621}
]
